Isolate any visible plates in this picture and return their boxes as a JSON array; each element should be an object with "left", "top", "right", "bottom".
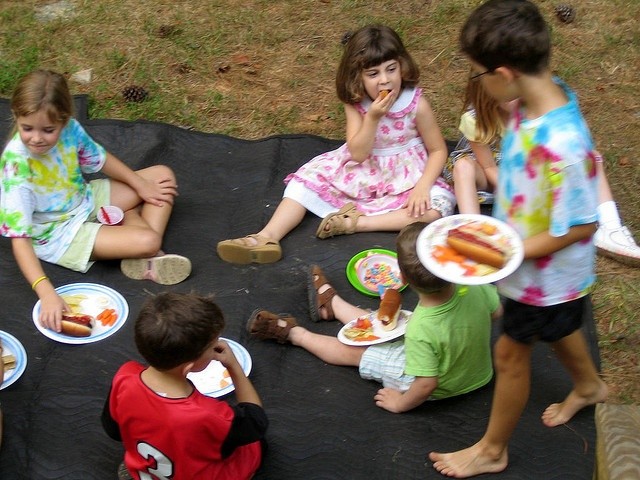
[
  {"left": 0, "top": 329, "right": 29, "bottom": 391},
  {"left": 32, "top": 282, "right": 129, "bottom": 345},
  {"left": 416, "top": 213, "right": 524, "bottom": 287},
  {"left": 186, "top": 336, "right": 252, "bottom": 400},
  {"left": 346, "top": 249, "right": 410, "bottom": 297},
  {"left": 338, "top": 310, "right": 413, "bottom": 347}
]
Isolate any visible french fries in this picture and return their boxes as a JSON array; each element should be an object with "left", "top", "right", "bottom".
[{"left": 98, "top": 309, "right": 117, "bottom": 326}]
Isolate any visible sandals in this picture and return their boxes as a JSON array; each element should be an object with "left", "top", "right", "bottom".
[
  {"left": 306, "top": 264, "right": 337, "bottom": 324},
  {"left": 245, "top": 309, "right": 299, "bottom": 343},
  {"left": 217, "top": 234, "right": 282, "bottom": 264},
  {"left": 593, "top": 226, "right": 640, "bottom": 268},
  {"left": 316, "top": 202, "right": 366, "bottom": 240},
  {"left": 120, "top": 254, "right": 191, "bottom": 286}
]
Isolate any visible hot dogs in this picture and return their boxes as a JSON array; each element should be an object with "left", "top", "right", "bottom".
[
  {"left": 378, "top": 289, "right": 402, "bottom": 329},
  {"left": 448, "top": 230, "right": 507, "bottom": 268},
  {"left": 60, "top": 313, "right": 95, "bottom": 337}
]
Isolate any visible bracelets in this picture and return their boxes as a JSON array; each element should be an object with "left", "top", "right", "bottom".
[{"left": 32, "top": 274, "right": 50, "bottom": 291}]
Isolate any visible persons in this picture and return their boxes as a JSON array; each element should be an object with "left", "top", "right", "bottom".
[
  {"left": 245, "top": 221, "right": 506, "bottom": 415},
  {"left": 428, "top": 0, "right": 610, "bottom": 480},
  {"left": 442, "top": 61, "right": 640, "bottom": 269},
  {"left": 100, "top": 293, "right": 270, "bottom": 480},
  {"left": 0, "top": 67, "right": 193, "bottom": 334},
  {"left": 215, "top": 24, "right": 458, "bottom": 266}
]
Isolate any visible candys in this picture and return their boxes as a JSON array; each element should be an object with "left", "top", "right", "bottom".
[{"left": 363, "top": 262, "right": 402, "bottom": 290}]
